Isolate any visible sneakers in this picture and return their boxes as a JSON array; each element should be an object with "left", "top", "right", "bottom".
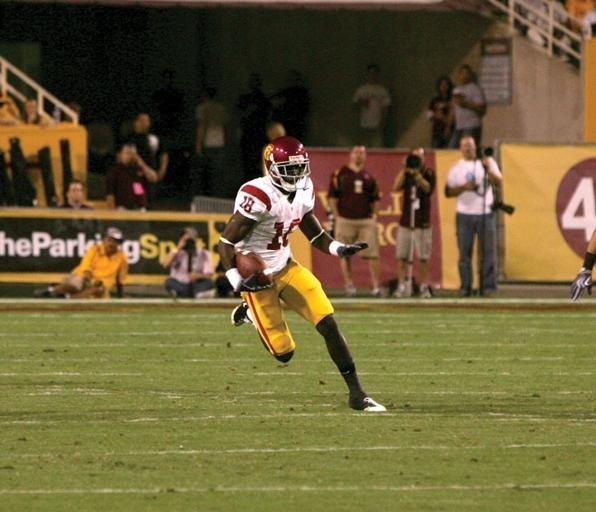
[
  {"left": 230, "top": 297, "right": 249, "bottom": 326},
  {"left": 348, "top": 392, "right": 387, "bottom": 411},
  {"left": 32, "top": 286, "right": 71, "bottom": 298},
  {"left": 345, "top": 283, "right": 431, "bottom": 298}
]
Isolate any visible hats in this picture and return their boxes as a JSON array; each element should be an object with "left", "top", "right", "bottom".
[
  {"left": 406, "top": 154, "right": 422, "bottom": 168},
  {"left": 105, "top": 226, "right": 123, "bottom": 240}
]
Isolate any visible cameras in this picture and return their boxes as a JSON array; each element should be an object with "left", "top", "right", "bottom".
[
  {"left": 405, "top": 153, "right": 423, "bottom": 171},
  {"left": 185, "top": 239, "right": 195, "bottom": 252},
  {"left": 474, "top": 144, "right": 495, "bottom": 158},
  {"left": 490, "top": 200, "right": 515, "bottom": 215}
]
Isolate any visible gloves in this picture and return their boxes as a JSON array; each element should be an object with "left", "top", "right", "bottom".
[
  {"left": 569, "top": 266, "right": 593, "bottom": 300},
  {"left": 337, "top": 243, "right": 368, "bottom": 258},
  {"left": 234, "top": 273, "right": 273, "bottom": 292}
]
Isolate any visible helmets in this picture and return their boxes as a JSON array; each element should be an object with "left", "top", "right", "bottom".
[{"left": 264, "top": 136, "right": 311, "bottom": 192}]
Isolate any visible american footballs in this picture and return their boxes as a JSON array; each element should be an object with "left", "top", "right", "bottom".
[{"left": 235, "top": 250, "right": 272, "bottom": 285}]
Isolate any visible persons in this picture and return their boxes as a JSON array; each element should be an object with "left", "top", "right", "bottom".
[
  {"left": 216, "top": 136, "right": 387, "bottom": 413},
  {"left": 165, "top": 227, "right": 241, "bottom": 297},
  {"left": 392, "top": 147, "right": 438, "bottom": 296},
  {"left": 236, "top": 70, "right": 309, "bottom": 180},
  {"left": 32, "top": 227, "right": 127, "bottom": 299},
  {"left": 353, "top": 65, "right": 391, "bottom": 147},
  {"left": 443, "top": 137, "right": 502, "bottom": 296},
  {"left": 151, "top": 69, "right": 229, "bottom": 193},
  {"left": 106, "top": 112, "right": 168, "bottom": 209},
  {"left": 426, "top": 66, "right": 484, "bottom": 148},
  {"left": 325, "top": 143, "right": 385, "bottom": 295},
  {"left": 26, "top": 98, "right": 89, "bottom": 208},
  {"left": 568, "top": 230, "right": 596, "bottom": 298},
  {"left": 480, "top": 1, "right": 596, "bottom": 61}
]
